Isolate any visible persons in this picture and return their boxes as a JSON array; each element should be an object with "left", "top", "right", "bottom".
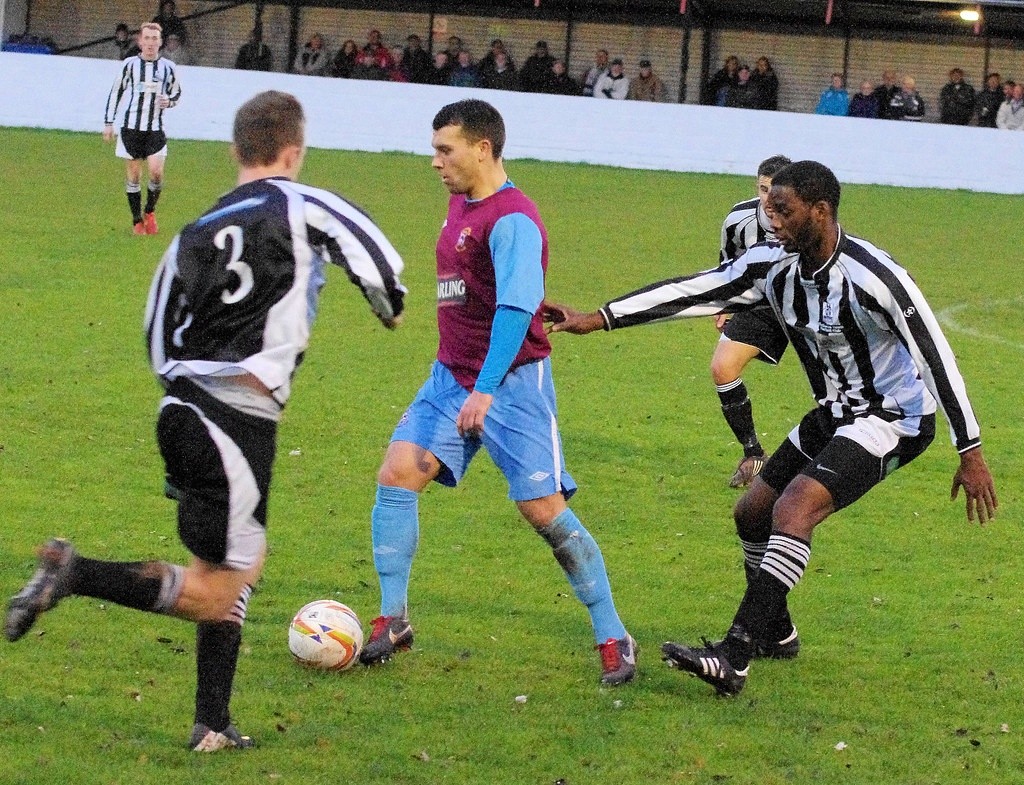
[
  {"left": 236, "top": 30, "right": 274, "bottom": 71},
  {"left": 103, "top": 21, "right": 181, "bottom": 235},
  {"left": 541, "top": 160, "right": 1000, "bottom": 697},
  {"left": 706, "top": 55, "right": 779, "bottom": 111},
  {"left": 106, "top": 0, "right": 189, "bottom": 64},
  {"left": 293, "top": 30, "right": 665, "bottom": 104},
  {"left": 361, "top": 100, "right": 641, "bottom": 689},
  {"left": 814, "top": 67, "right": 1024, "bottom": 132},
  {"left": 710, "top": 154, "right": 796, "bottom": 488},
  {"left": 2, "top": 91, "right": 409, "bottom": 752}
]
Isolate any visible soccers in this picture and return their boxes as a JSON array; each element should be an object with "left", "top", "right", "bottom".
[{"left": 287, "top": 599, "right": 364, "bottom": 671}]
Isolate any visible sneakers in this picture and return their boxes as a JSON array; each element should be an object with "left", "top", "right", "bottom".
[
  {"left": 663, "top": 637, "right": 749, "bottom": 695},
  {"left": 187, "top": 723, "right": 253, "bottom": 752},
  {"left": 728, "top": 449, "right": 769, "bottom": 486},
  {"left": 712, "top": 625, "right": 799, "bottom": 658},
  {"left": 10, "top": 539, "right": 70, "bottom": 641},
  {"left": 599, "top": 630, "right": 640, "bottom": 686},
  {"left": 360, "top": 615, "right": 413, "bottom": 665},
  {"left": 134, "top": 217, "right": 145, "bottom": 234},
  {"left": 144, "top": 212, "right": 157, "bottom": 234}
]
